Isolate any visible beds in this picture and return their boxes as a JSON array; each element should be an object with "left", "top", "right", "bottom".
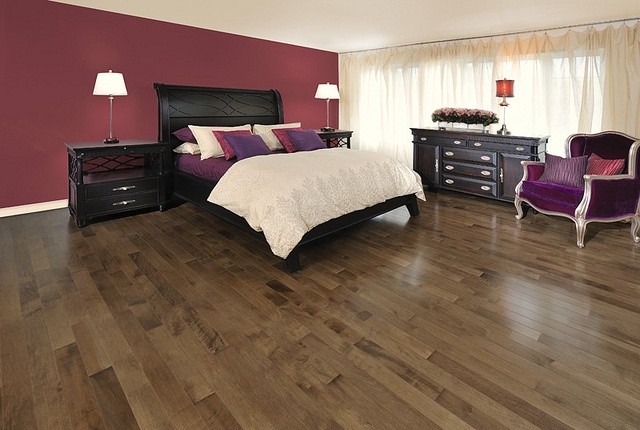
[{"left": 153, "top": 82, "right": 427, "bottom": 272}]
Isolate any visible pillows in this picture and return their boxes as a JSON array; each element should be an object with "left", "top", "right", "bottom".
[
  {"left": 286, "top": 130, "right": 327, "bottom": 151},
  {"left": 272, "top": 127, "right": 304, "bottom": 153},
  {"left": 212, "top": 130, "right": 252, "bottom": 161},
  {"left": 171, "top": 127, "right": 198, "bottom": 144},
  {"left": 172, "top": 142, "right": 201, "bottom": 155},
  {"left": 253, "top": 122, "right": 302, "bottom": 151},
  {"left": 536, "top": 152, "right": 589, "bottom": 188},
  {"left": 188, "top": 124, "right": 252, "bottom": 161},
  {"left": 224, "top": 135, "right": 272, "bottom": 160},
  {"left": 586, "top": 152, "right": 625, "bottom": 176}
]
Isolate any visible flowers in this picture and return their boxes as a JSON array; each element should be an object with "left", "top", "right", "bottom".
[{"left": 431, "top": 107, "right": 500, "bottom": 127}]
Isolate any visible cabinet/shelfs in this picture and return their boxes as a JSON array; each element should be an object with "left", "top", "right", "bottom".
[{"left": 410, "top": 128, "right": 551, "bottom": 208}]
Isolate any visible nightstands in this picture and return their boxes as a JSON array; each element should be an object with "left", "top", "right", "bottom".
[
  {"left": 63, "top": 139, "right": 167, "bottom": 227},
  {"left": 317, "top": 130, "right": 354, "bottom": 149}
]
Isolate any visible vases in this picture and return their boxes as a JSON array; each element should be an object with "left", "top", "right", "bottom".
[{"left": 438, "top": 121, "right": 489, "bottom": 133}]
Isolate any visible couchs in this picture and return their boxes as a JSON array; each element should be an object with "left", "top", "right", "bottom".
[{"left": 514, "top": 131, "right": 640, "bottom": 248}]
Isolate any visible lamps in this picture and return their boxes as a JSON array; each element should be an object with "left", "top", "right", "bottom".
[
  {"left": 314, "top": 82, "right": 340, "bottom": 132},
  {"left": 93, "top": 70, "right": 128, "bottom": 143},
  {"left": 495, "top": 78, "right": 515, "bottom": 136}
]
